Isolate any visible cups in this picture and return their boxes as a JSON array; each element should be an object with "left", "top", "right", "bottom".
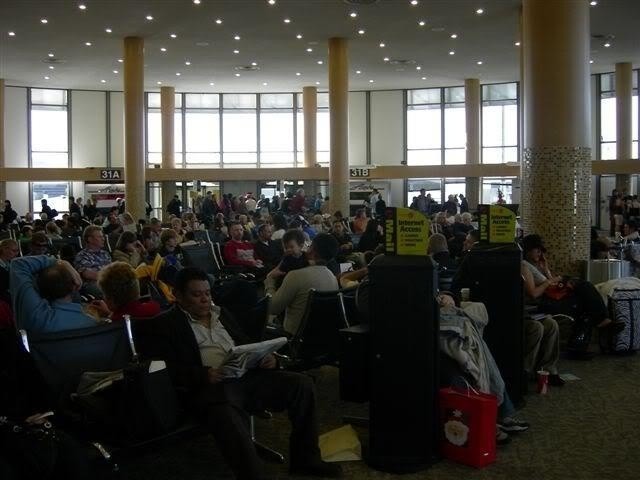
[
  {"left": 460, "top": 288, "right": 470, "bottom": 301},
  {"left": 536, "top": 371, "right": 550, "bottom": 394}
]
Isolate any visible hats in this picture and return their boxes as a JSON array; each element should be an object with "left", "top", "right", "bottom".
[{"left": 523, "top": 234, "right": 547, "bottom": 254}]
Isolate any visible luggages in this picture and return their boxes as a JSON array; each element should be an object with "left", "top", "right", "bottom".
[{"left": 595, "top": 245, "right": 640, "bottom": 354}]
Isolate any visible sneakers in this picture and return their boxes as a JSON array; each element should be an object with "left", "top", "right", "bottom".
[
  {"left": 496, "top": 418, "right": 530, "bottom": 433},
  {"left": 494, "top": 427, "right": 512, "bottom": 447}
]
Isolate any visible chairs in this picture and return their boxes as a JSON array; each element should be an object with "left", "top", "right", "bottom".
[
  {"left": 50, "top": 237, "right": 82, "bottom": 255},
  {"left": 216, "top": 242, "right": 225, "bottom": 269},
  {"left": 272, "top": 284, "right": 358, "bottom": 374},
  {"left": 181, "top": 242, "right": 222, "bottom": 276},
  {"left": 126, "top": 305, "right": 285, "bottom": 464},
  {"left": 192, "top": 229, "right": 210, "bottom": 244},
  {"left": 20, "top": 313, "right": 170, "bottom": 472},
  {"left": 18, "top": 238, "right": 32, "bottom": 258},
  {"left": 79, "top": 234, "right": 112, "bottom": 253}
]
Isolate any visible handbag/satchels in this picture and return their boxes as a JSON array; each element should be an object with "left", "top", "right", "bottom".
[
  {"left": 319, "top": 424, "right": 362, "bottom": 462},
  {"left": 437, "top": 375, "right": 496, "bottom": 468},
  {"left": 551, "top": 274, "right": 582, "bottom": 302},
  {"left": 0, "top": 415, "right": 71, "bottom": 474}
]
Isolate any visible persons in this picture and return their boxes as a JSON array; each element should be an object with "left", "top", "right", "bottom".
[
  {"left": 409, "top": 188, "right": 475, "bottom": 228},
  {"left": 353, "top": 209, "right": 369, "bottom": 234},
  {"left": 260, "top": 233, "right": 340, "bottom": 357},
  {"left": 144, "top": 266, "right": 343, "bottom": 480},
  {"left": 90, "top": 260, "right": 160, "bottom": 325},
  {"left": 333, "top": 211, "right": 353, "bottom": 234},
  {"left": 520, "top": 233, "right": 626, "bottom": 361},
  {"left": 363, "top": 188, "right": 387, "bottom": 224},
  {"left": 457, "top": 229, "right": 480, "bottom": 269},
  {"left": 170, "top": 217, "right": 185, "bottom": 246},
  {"left": 0, "top": 238, "right": 20, "bottom": 305},
  {"left": 330, "top": 220, "right": 364, "bottom": 265},
  {"left": 525, "top": 315, "right": 567, "bottom": 386},
  {"left": 25, "top": 232, "right": 58, "bottom": 256},
  {"left": 223, "top": 221, "right": 265, "bottom": 283},
  {"left": 166, "top": 194, "right": 182, "bottom": 218},
  {"left": 427, "top": 233, "right": 453, "bottom": 278},
  {"left": 183, "top": 187, "right": 333, "bottom": 236},
  {"left": 72, "top": 224, "right": 113, "bottom": 301},
  {"left": 103, "top": 213, "right": 124, "bottom": 252},
  {"left": 9, "top": 253, "right": 119, "bottom": 399},
  {"left": 338, "top": 267, "right": 370, "bottom": 309},
  {"left": 119, "top": 212, "right": 138, "bottom": 234},
  {"left": 436, "top": 290, "right": 531, "bottom": 449},
  {"left": 0, "top": 195, "right": 97, "bottom": 242},
  {"left": 113, "top": 230, "right": 153, "bottom": 296},
  {"left": 265, "top": 229, "right": 309, "bottom": 330},
  {"left": 609, "top": 186, "right": 640, "bottom": 243},
  {"left": 156, "top": 228, "right": 215, "bottom": 290},
  {"left": 357, "top": 218, "right": 385, "bottom": 253},
  {"left": 254, "top": 224, "right": 284, "bottom": 274}
]
